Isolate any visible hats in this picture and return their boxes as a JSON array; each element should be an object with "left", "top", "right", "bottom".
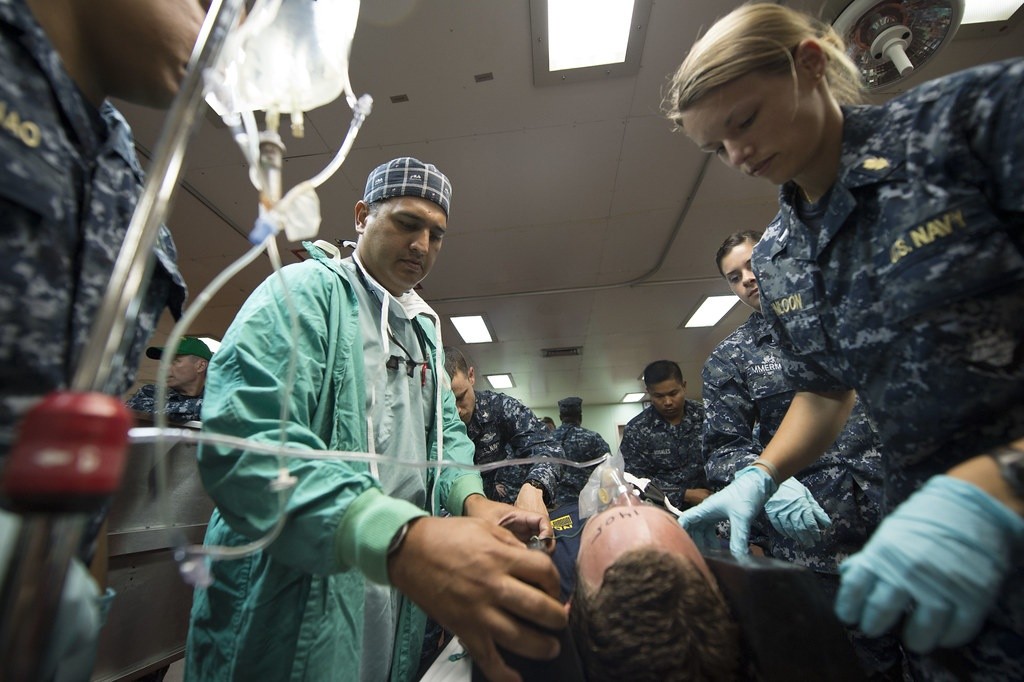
[
  {"left": 557, "top": 397, "right": 584, "bottom": 412},
  {"left": 362, "top": 157, "right": 453, "bottom": 218},
  {"left": 146, "top": 338, "right": 213, "bottom": 359}
]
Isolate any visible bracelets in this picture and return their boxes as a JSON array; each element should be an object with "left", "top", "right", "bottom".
[{"left": 750, "top": 458, "right": 781, "bottom": 485}]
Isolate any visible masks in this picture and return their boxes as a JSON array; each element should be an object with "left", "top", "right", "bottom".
[{"left": 342, "top": 239, "right": 435, "bottom": 321}]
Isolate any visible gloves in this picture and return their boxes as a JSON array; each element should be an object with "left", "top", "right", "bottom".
[
  {"left": 676, "top": 465, "right": 778, "bottom": 560},
  {"left": 765, "top": 474, "right": 832, "bottom": 551},
  {"left": 833, "top": 475, "right": 1024, "bottom": 653}
]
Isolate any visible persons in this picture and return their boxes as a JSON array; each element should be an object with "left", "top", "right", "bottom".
[
  {"left": 180, "top": 157, "right": 574, "bottom": 682},
  {"left": 441, "top": 227, "right": 915, "bottom": 682},
  {"left": 0, "top": 0, "right": 246, "bottom": 682},
  {"left": 658, "top": 3, "right": 1024, "bottom": 682},
  {"left": 124, "top": 337, "right": 213, "bottom": 427}
]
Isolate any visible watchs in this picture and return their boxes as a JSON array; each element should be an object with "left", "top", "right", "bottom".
[
  {"left": 389, "top": 521, "right": 408, "bottom": 555},
  {"left": 985, "top": 443, "right": 1024, "bottom": 500}
]
